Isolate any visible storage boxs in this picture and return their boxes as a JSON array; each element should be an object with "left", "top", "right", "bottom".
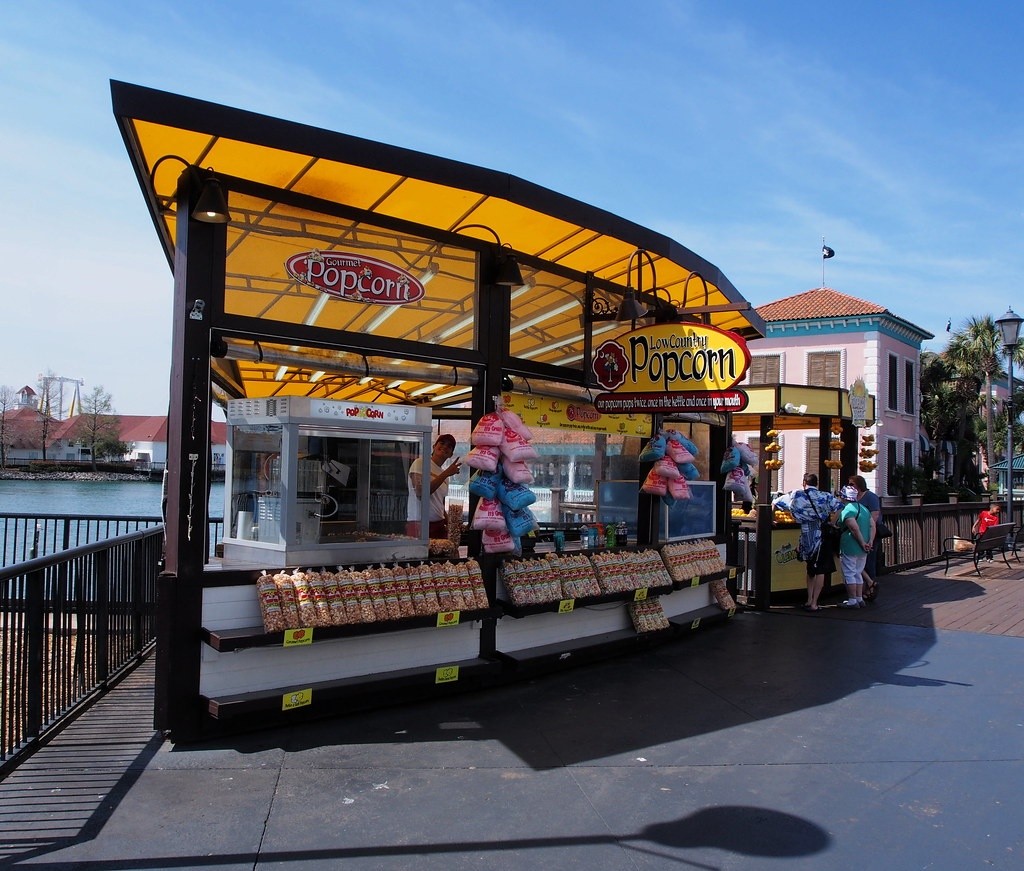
[
  {"left": 221, "top": 395, "right": 434, "bottom": 568},
  {"left": 592, "top": 477, "right": 718, "bottom": 544}
]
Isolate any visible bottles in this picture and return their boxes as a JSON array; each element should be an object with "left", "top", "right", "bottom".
[{"left": 552, "top": 522, "right": 629, "bottom": 552}]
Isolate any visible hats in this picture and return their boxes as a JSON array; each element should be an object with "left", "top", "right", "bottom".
[
  {"left": 436, "top": 434, "right": 456, "bottom": 446},
  {"left": 838, "top": 485, "right": 858, "bottom": 503}
]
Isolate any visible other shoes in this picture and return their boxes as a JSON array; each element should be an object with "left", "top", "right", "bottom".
[
  {"left": 802, "top": 603, "right": 824, "bottom": 612},
  {"left": 978, "top": 558, "right": 994, "bottom": 564},
  {"left": 839, "top": 581, "right": 879, "bottom": 608}
]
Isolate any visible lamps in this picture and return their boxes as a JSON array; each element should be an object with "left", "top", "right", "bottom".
[
  {"left": 150, "top": 154, "right": 231, "bottom": 226},
  {"left": 450, "top": 223, "right": 524, "bottom": 287},
  {"left": 616, "top": 248, "right": 668, "bottom": 323}
]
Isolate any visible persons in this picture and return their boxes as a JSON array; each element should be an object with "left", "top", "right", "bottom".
[
  {"left": 847, "top": 475, "right": 883, "bottom": 601},
  {"left": 790, "top": 474, "right": 842, "bottom": 609},
  {"left": 404, "top": 432, "right": 464, "bottom": 541},
  {"left": 836, "top": 483, "right": 877, "bottom": 609}
]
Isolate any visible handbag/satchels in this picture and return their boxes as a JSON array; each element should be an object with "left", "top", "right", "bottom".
[
  {"left": 821, "top": 523, "right": 840, "bottom": 552},
  {"left": 876, "top": 524, "right": 892, "bottom": 538}
]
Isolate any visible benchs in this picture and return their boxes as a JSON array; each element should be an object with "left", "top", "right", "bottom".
[
  {"left": 1005, "top": 522, "right": 1024, "bottom": 563},
  {"left": 941, "top": 521, "right": 1017, "bottom": 578}
]
